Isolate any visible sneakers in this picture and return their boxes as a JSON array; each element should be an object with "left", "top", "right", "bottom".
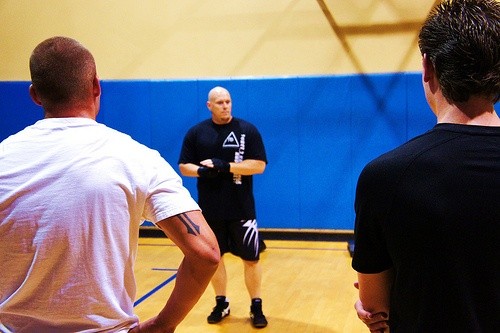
[
  {"left": 207, "top": 296, "right": 231, "bottom": 324},
  {"left": 250, "top": 298, "right": 268, "bottom": 328}
]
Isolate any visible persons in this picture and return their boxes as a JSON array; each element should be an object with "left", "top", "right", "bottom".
[
  {"left": 0, "top": 37, "right": 222, "bottom": 333},
  {"left": 352, "top": 0, "right": 500, "bottom": 333},
  {"left": 178, "top": 86, "right": 268, "bottom": 328}
]
